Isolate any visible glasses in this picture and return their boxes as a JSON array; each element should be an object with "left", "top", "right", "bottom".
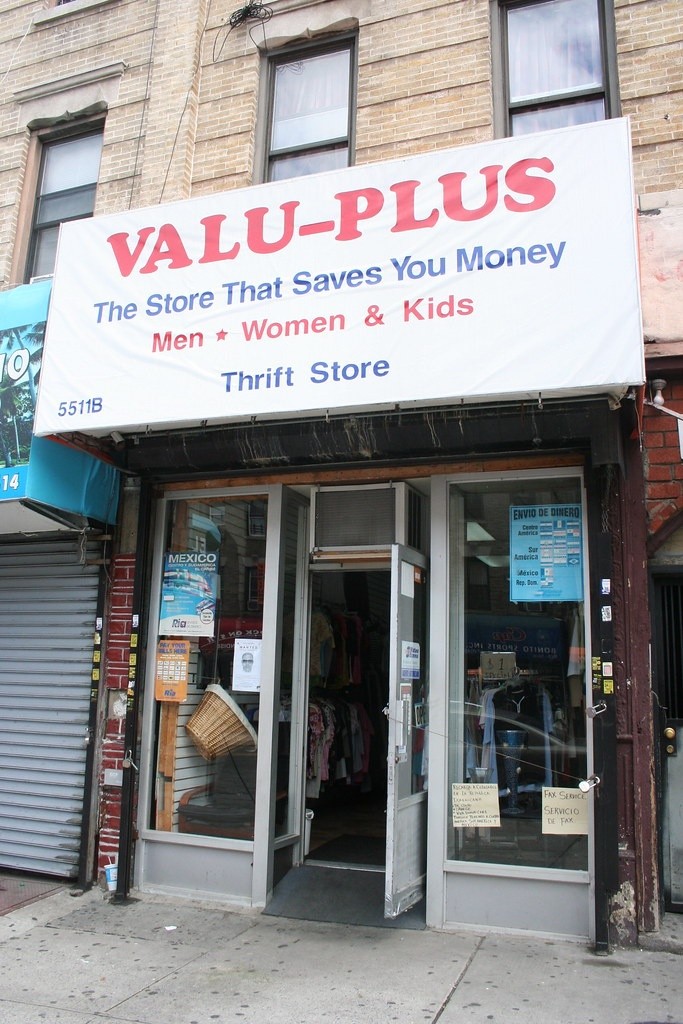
[{"left": 243, "top": 660, "right": 253, "bottom": 663}]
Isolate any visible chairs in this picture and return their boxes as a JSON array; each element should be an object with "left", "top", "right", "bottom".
[{"left": 178, "top": 747, "right": 289, "bottom": 839}]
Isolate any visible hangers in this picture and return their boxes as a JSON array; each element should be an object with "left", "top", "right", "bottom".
[{"left": 508, "top": 667, "right": 526, "bottom": 693}]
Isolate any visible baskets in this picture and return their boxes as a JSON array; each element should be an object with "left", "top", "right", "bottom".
[{"left": 186, "top": 678, "right": 257, "bottom": 762}]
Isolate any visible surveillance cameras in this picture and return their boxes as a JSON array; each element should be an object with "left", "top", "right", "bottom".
[{"left": 111, "top": 432, "right": 125, "bottom": 446}]
